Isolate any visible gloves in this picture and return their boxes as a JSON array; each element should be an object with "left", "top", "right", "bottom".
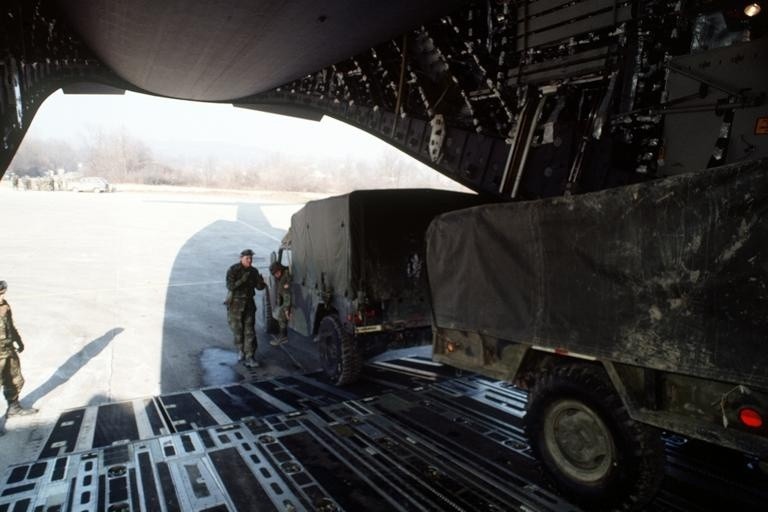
[{"left": 241, "top": 272, "right": 250, "bottom": 283}]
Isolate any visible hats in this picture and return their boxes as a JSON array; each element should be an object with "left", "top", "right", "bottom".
[
  {"left": 270, "top": 262, "right": 283, "bottom": 275},
  {"left": 241, "top": 250, "right": 253, "bottom": 256}
]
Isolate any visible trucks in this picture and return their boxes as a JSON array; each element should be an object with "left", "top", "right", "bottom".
[
  {"left": 68, "top": 177, "right": 108, "bottom": 193},
  {"left": 259, "top": 187, "right": 485, "bottom": 388},
  {"left": 423, "top": 39, "right": 766, "bottom": 510}
]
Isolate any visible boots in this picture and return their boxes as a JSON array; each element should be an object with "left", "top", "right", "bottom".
[
  {"left": 238, "top": 350, "right": 259, "bottom": 367},
  {"left": 270, "top": 328, "right": 288, "bottom": 345},
  {"left": 5, "top": 397, "right": 39, "bottom": 418}
]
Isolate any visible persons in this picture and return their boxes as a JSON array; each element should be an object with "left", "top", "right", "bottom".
[
  {"left": 267, "top": 261, "right": 293, "bottom": 347},
  {"left": 223, "top": 248, "right": 268, "bottom": 368},
  {"left": 0, "top": 279, "right": 41, "bottom": 438}
]
